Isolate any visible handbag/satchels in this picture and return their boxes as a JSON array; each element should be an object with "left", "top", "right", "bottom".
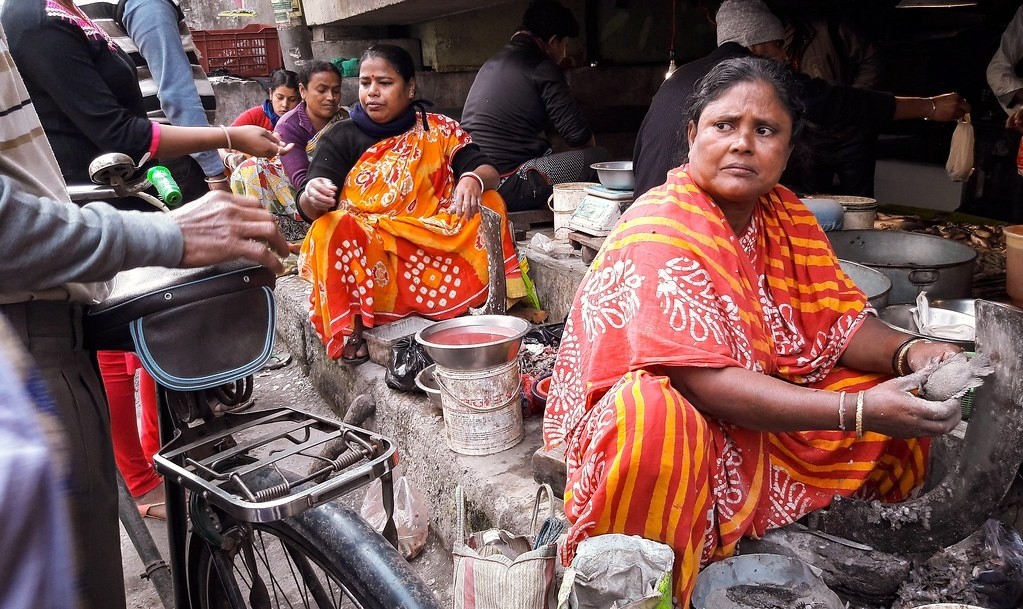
[
  {"left": 451, "top": 483, "right": 563, "bottom": 609},
  {"left": 361, "top": 465, "right": 427, "bottom": 561}
]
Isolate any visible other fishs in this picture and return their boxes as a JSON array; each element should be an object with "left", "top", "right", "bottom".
[
  {"left": 920, "top": 351, "right": 996, "bottom": 407},
  {"left": 726, "top": 584, "right": 799, "bottom": 609}
]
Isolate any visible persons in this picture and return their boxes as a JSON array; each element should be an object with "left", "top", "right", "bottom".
[
  {"left": 229, "top": 59, "right": 350, "bottom": 241},
  {"left": 775, "top": 11, "right": 843, "bottom": 193},
  {"left": 296, "top": 44, "right": 528, "bottom": 364},
  {"left": 986, "top": 3, "right": 1023, "bottom": 224},
  {"left": 1, "top": 0, "right": 296, "bottom": 519},
  {"left": 217, "top": 68, "right": 302, "bottom": 180},
  {"left": 542, "top": 57, "right": 970, "bottom": 608},
  {"left": 0, "top": 18, "right": 289, "bottom": 609},
  {"left": 632, "top": 0, "right": 972, "bottom": 199},
  {"left": 72, "top": 0, "right": 253, "bottom": 416},
  {"left": 460, "top": 0, "right": 614, "bottom": 213}
]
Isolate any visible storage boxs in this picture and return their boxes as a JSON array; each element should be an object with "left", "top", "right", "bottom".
[
  {"left": 361, "top": 316, "right": 435, "bottom": 366},
  {"left": 183, "top": 22, "right": 282, "bottom": 77}
]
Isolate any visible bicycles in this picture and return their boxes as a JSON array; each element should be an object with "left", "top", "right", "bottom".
[{"left": 65, "top": 151, "right": 444, "bottom": 609}]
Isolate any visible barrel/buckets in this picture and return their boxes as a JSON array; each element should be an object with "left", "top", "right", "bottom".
[
  {"left": 433, "top": 356, "right": 524, "bottom": 456},
  {"left": 825, "top": 230, "right": 978, "bottom": 306},
  {"left": 548, "top": 182, "right": 601, "bottom": 240},
  {"left": 837, "top": 259, "right": 892, "bottom": 311},
  {"left": 1002, "top": 225, "right": 1023, "bottom": 301},
  {"left": 806, "top": 195, "right": 877, "bottom": 230}
]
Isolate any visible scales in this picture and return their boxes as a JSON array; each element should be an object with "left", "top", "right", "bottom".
[{"left": 568, "top": 186, "right": 636, "bottom": 237}]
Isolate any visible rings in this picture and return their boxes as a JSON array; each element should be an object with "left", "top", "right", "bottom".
[{"left": 275, "top": 146, "right": 281, "bottom": 156}]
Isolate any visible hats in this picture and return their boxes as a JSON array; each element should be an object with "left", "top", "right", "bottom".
[
  {"left": 525, "top": 1, "right": 580, "bottom": 39},
  {"left": 716, "top": 0, "right": 786, "bottom": 48}
]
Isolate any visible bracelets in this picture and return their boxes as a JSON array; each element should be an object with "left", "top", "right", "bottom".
[
  {"left": 892, "top": 336, "right": 932, "bottom": 376},
  {"left": 460, "top": 172, "right": 484, "bottom": 194},
  {"left": 855, "top": 389, "right": 865, "bottom": 439},
  {"left": 224, "top": 153, "right": 236, "bottom": 169},
  {"left": 838, "top": 391, "right": 847, "bottom": 431},
  {"left": 922, "top": 96, "right": 935, "bottom": 120},
  {"left": 219, "top": 125, "right": 231, "bottom": 153},
  {"left": 204, "top": 175, "right": 230, "bottom": 183}
]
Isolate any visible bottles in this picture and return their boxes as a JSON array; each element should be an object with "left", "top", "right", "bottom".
[{"left": 482, "top": 530, "right": 517, "bottom": 561}]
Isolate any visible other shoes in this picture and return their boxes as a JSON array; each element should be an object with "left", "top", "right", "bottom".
[{"left": 206, "top": 394, "right": 253, "bottom": 414}]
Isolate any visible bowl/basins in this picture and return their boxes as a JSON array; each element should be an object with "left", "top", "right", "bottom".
[
  {"left": 878, "top": 304, "right": 976, "bottom": 344},
  {"left": 590, "top": 161, "right": 635, "bottom": 190},
  {"left": 415, "top": 315, "right": 533, "bottom": 370},
  {"left": 931, "top": 299, "right": 1022, "bottom": 318},
  {"left": 531, "top": 372, "right": 553, "bottom": 411},
  {"left": 415, "top": 365, "right": 443, "bottom": 410}
]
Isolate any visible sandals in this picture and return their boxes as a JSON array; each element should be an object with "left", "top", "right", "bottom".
[
  {"left": 260, "top": 352, "right": 292, "bottom": 370},
  {"left": 135, "top": 487, "right": 191, "bottom": 521},
  {"left": 340, "top": 331, "right": 370, "bottom": 365}
]
